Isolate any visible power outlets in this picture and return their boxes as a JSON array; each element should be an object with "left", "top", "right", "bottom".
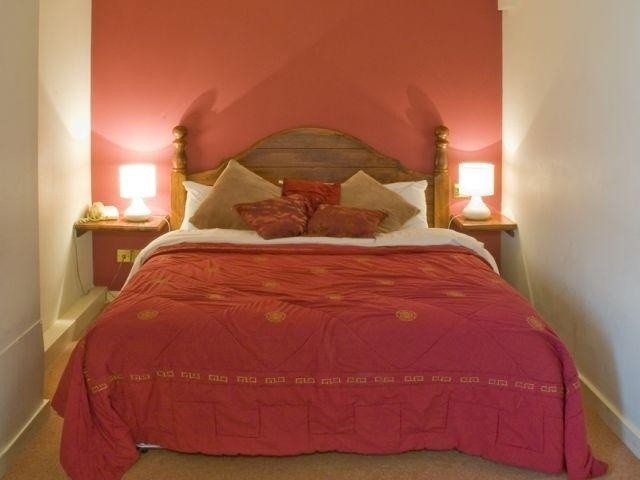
[{"left": 116, "top": 249, "right": 131, "bottom": 263}]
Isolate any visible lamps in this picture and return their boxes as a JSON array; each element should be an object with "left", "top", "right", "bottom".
[
  {"left": 118, "top": 162, "right": 157, "bottom": 222},
  {"left": 457, "top": 160, "right": 495, "bottom": 219}
]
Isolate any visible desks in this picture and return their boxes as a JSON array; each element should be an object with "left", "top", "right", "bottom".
[
  {"left": 450, "top": 213, "right": 518, "bottom": 233},
  {"left": 75, "top": 215, "right": 169, "bottom": 233}
]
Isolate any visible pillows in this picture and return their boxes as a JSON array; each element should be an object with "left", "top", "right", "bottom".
[
  {"left": 341, "top": 170, "right": 421, "bottom": 234},
  {"left": 301, "top": 203, "right": 389, "bottom": 238},
  {"left": 180, "top": 181, "right": 238, "bottom": 231},
  {"left": 278, "top": 177, "right": 428, "bottom": 231},
  {"left": 234, "top": 194, "right": 313, "bottom": 239},
  {"left": 188, "top": 157, "right": 282, "bottom": 231},
  {"left": 282, "top": 175, "right": 341, "bottom": 212}
]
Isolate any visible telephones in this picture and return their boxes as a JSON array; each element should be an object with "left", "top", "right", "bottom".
[{"left": 88, "top": 202, "right": 119, "bottom": 221}]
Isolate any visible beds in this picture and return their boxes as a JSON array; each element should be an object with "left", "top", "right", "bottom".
[{"left": 49, "top": 124, "right": 608, "bottom": 480}]
